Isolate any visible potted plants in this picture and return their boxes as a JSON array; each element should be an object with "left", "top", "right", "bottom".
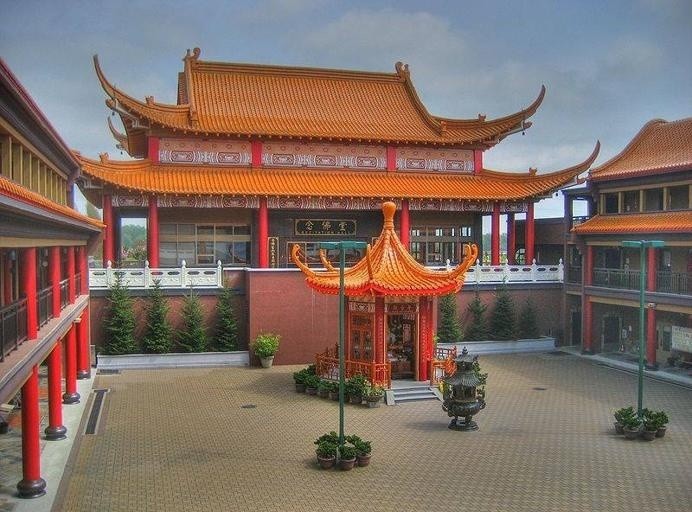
[
  {"left": 248, "top": 328, "right": 283, "bottom": 368},
  {"left": 314, "top": 431, "right": 374, "bottom": 471},
  {"left": 614, "top": 406, "right": 669, "bottom": 441},
  {"left": 292, "top": 362, "right": 386, "bottom": 408}
]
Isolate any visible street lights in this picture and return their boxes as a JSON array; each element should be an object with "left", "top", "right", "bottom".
[
  {"left": 621, "top": 241, "right": 665, "bottom": 415},
  {"left": 317, "top": 242, "right": 367, "bottom": 448}
]
